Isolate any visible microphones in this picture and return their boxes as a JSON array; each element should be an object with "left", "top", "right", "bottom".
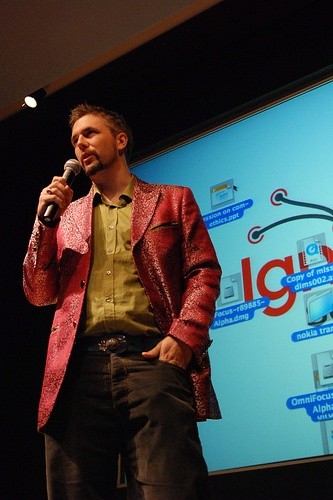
[{"left": 42, "top": 159, "right": 81, "bottom": 223}]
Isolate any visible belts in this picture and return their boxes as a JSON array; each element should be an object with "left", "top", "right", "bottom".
[{"left": 71, "top": 331, "right": 161, "bottom": 356}]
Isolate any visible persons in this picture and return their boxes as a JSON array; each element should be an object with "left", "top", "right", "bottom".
[{"left": 22, "top": 104, "right": 222, "bottom": 500}]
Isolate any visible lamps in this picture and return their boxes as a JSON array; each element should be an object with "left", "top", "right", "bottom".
[{"left": 25, "top": 89, "right": 46, "bottom": 109}]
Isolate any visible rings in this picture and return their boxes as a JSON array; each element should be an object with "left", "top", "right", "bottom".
[{"left": 47, "top": 189, "right": 52, "bottom": 194}]
